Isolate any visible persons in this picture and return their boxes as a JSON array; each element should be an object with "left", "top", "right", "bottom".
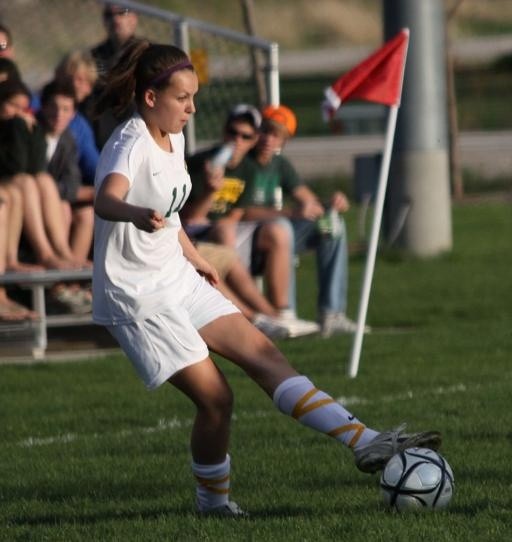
[
  {"left": 229, "top": 103, "right": 375, "bottom": 337},
  {"left": 0, "top": 4, "right": 138, "bottom": 325},
  {"left": 188, "top": 102, "right": 320, "bottom": 338},
  {"left": 90, "top": 40, "right": 443, "bottom": 522}
]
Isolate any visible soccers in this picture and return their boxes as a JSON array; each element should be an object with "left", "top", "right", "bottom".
[{"left": 379, "top": 446, "right": 455, "bottom": 511}]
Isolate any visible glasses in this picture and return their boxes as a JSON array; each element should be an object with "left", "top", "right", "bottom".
[
  {"left": 104, "top": 6, "right": 131, "bottom": 17},
  {"left": 226, "top": 125, "right": 253, "bottom": 140}
]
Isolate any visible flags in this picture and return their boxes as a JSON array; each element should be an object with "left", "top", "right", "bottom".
[{"left": 324, "top": 29, "right": 409, "bottom": 121}]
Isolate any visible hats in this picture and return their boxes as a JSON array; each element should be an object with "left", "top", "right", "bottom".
[
  {"left": 262, "top": 104, "right": 300, "bottom": 136},
  {"left": 229, "top": 103, "right": 262, "bottom": 130}
]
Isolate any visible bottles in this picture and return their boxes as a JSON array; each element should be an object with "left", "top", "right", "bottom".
[{"left": 211, "top": 138, "right": 236, "bottom": 168}]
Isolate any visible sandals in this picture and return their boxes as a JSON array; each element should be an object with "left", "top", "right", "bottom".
[{"left": 0, "top": 281, "right": 93, "bottom": 325}]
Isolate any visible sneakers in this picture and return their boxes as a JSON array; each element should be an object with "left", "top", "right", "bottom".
[
  {"left": 318, "top": 313, "right": 374, "bottom": 338},
  {"left": 253, "top": 315, "right": 290, "bottom": 341},
  {"left": 279, "top": 310, "right": 319, "bottom": 338},
  {"left": 354, "top": 426, "right": 444, "bottom": 475}
]
